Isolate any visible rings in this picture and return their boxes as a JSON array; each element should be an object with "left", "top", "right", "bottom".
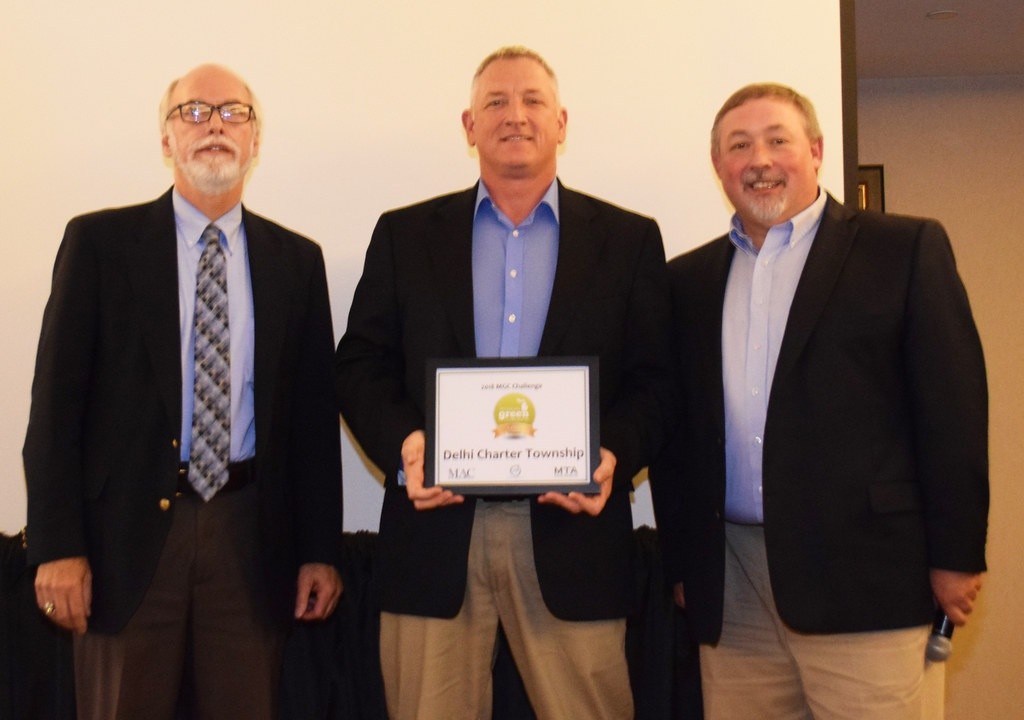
[{"left": 44, "top": 602, "right": 55, "bottom": 615}]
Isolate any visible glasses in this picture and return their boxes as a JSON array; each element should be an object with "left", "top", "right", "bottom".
[{"left": 166, "top": 101, "right": 256, "bottom": 124}]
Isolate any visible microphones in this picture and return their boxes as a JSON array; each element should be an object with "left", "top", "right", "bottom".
[{"left": 928, "top": 611, "right": 955, "bottom": 661}]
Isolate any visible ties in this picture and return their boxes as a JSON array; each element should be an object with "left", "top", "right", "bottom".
[{"left": 187, "top": 223, "right": 231, "bottom": 501}]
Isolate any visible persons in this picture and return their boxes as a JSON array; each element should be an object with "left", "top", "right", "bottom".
[
  {"left": 336, "top": 45, "right": 682, "bottom": 720},
  {"left": 666, "top": 85, "right": 990, "bottom": 720},
  {"left": 23, "top": 63, "right": 343, "bottom": 720}
]
